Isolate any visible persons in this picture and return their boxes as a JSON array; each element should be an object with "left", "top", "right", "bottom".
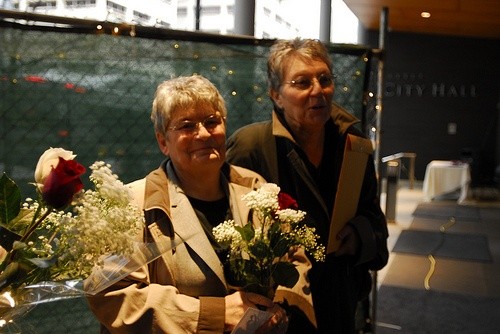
[
  {"left": 225, "top": 38, "right": 389, "bottom": 334},
  {"left": 86, "top": 74, "right": 317, "bottom": 334}
]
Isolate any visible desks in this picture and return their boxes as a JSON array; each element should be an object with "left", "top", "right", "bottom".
[{"left": 423, "top": 160, "right": 472, "bottom": 203}]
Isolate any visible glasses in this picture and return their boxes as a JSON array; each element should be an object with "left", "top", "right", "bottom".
[
  {"left": 279, "top": 73, "right": 337, "bottom": 88},
  {"left": 163, "top": 114, "right": 226, "bottom": 134}
]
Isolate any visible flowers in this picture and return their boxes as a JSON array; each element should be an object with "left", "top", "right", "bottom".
[
  {"left": 212, "top": 183, "right": 328, "bottom": 314},
  {"left": 0, "top": 147, "right": 145, "bottom": 328}
]
